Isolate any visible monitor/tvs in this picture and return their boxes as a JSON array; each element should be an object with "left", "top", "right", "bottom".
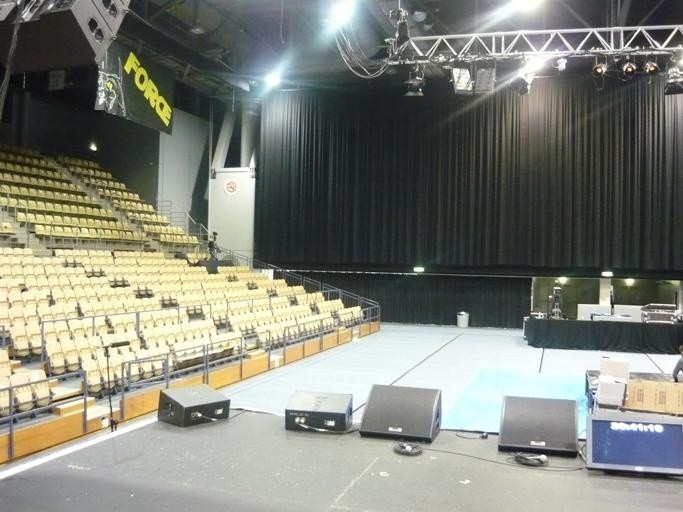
[{"left": 586, "top": 414, "right": 683, "bottom": 476}]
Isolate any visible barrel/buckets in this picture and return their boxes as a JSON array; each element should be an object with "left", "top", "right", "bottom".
[{"left": 457, "top": 311, "right": 469, "bottom": 328}]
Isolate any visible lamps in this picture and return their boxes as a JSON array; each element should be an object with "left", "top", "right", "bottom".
[{"left": 403, "top": 49, "right": 683, "bottom": 94}]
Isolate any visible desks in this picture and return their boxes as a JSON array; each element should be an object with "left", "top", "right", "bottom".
[{"left": 526, "top": 316, "right": 683, "bottom": 353}]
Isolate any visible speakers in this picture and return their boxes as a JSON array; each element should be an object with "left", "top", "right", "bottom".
[
  {"left": 285, "top": 390, "right": 353, "bottom": 433},
  {"left": 158, "top": 383, "right": 230, "bottom": 427},
  {"left": 359, "top": 385, "right": 442, "bottom": 443},
  {"left": 498, "top": 396, "right": 578, "bottom": 458},
  {"left": 0, "top": 0, "right": 131, "bottom": 73}
]
